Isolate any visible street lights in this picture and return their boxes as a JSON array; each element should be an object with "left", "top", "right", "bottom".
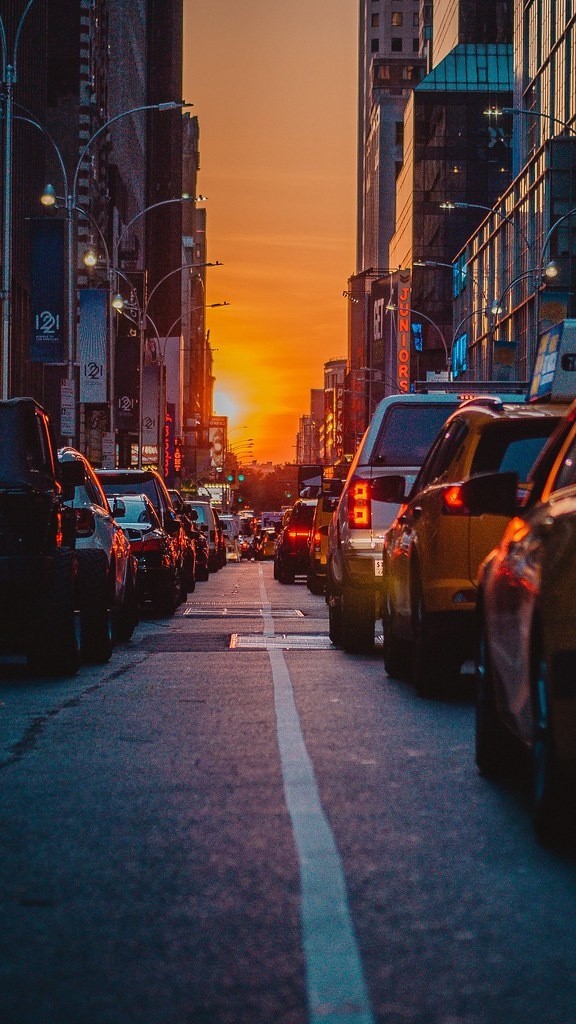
[
  {"left": 40, "top": 181, "right": 210, "bottom": 473},
  {"left": 412, "top": 259, "right": 563, "bottom": 393},
  {"left": 386, "top": 297, "right": 504, "bottom": 395},
  {"left": 82, "top": 248, "right": 225, "bottom": 473},
  {"left": 0, "top": 90, "right": 196, "bottom": 460},
  {"left": 438, "top": 200, "right": 576, "bottom": 356},
  {"left": 112, "top": 292, "right": 233, "bottom": 484}
]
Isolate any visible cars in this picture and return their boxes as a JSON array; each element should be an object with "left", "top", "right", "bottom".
[
  {"left": 105, "top": 490, "right": 189, "bottom": 615},
  {"left": 91, "top": 467, "right": 347, "bottom": 596},
  {"left": 458, "top": 392, "right": 576, "bottom": 854}
]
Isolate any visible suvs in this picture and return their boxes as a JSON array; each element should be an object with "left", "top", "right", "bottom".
[
  {"left": 362, "top": 393, "right": 573, "bottom": 698},
  {"left": 0, "top": 396, "right": 96, "bottom": 679},
  {"left": 55, "top": 444, "right": 139, "bottom": 665},
  {"left": 293, "top": 390, "right": 533, "bottom": 652}
]
